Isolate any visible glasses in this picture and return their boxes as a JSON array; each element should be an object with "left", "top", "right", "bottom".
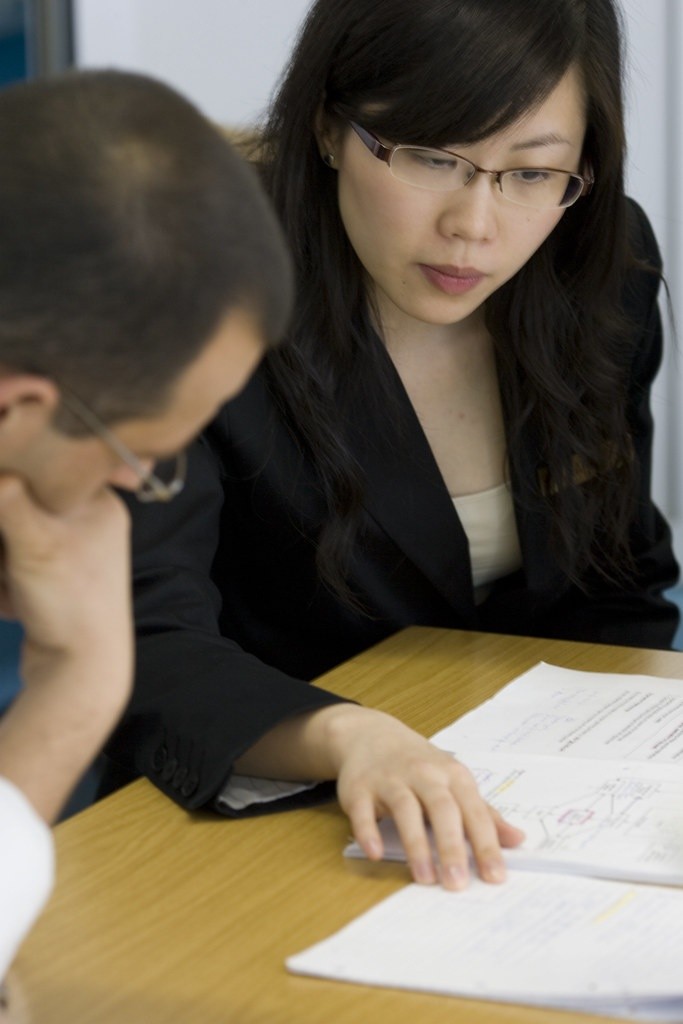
[
  {"left": 336, "top": 98, "right": 595, "bottom": 210},
  {"left": 59, "top": 383, "right": 188, "bottom": 503}
]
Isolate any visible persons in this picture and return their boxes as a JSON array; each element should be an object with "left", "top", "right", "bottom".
[
  {"left": 114, "top": 0, "right": 682, "bottom": 892},
  {"left": 0, "top": 70, "right": 290, "bottom": 999}
]
